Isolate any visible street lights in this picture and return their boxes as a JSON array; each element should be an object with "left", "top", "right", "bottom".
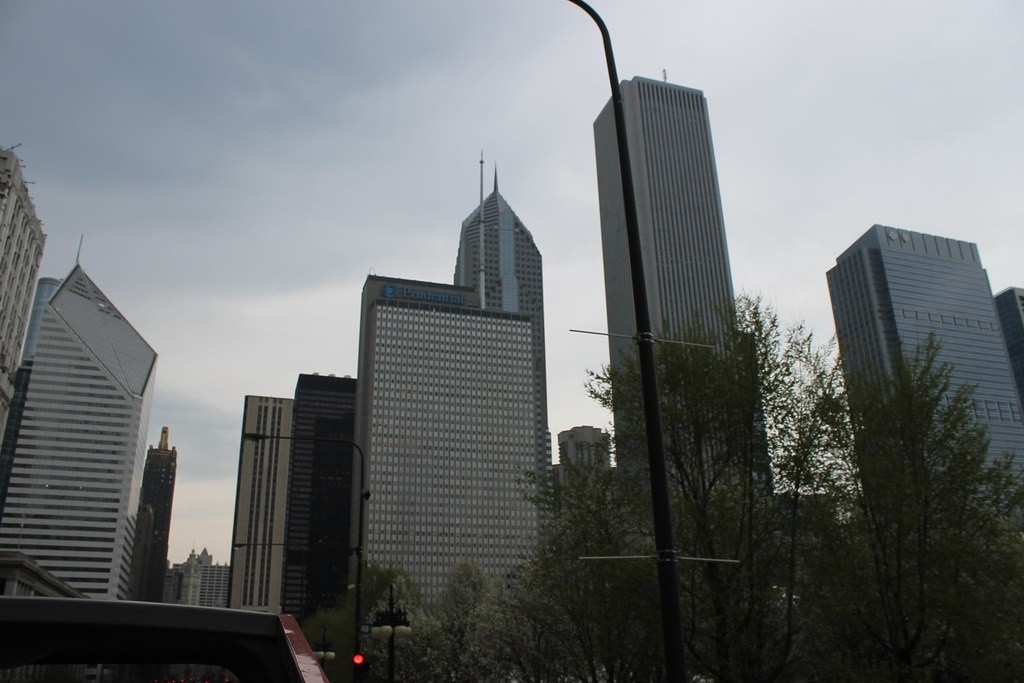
[
  {"left": 369, "top": 581, "right": 413, "bottom": 683},
  {"left": 311, "top": 628, "right": 336, "bottom": 671},
  {"left": 243, "top": 432, "right": 372, "bottom": 683}
]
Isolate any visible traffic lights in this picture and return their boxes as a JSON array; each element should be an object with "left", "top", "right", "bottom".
[{"left": 353, "top": 649, "right": 369, "bottom": 673}]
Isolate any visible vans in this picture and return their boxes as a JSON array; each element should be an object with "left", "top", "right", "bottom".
[{"left": 0, "top": 595, "right": 331, "bottom": 683}]
